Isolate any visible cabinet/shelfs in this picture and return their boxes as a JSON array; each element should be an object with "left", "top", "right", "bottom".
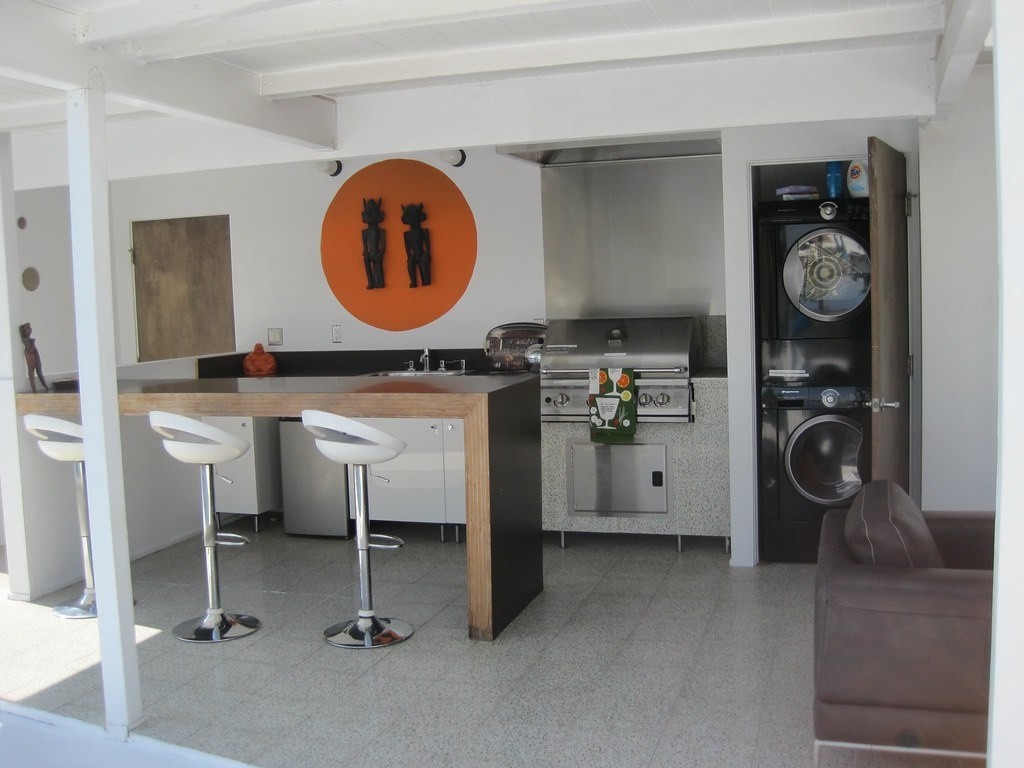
[
  {"left": 201, "top": 415, "right": 281, "bottom": 533},
  {"left": 348, "top": 419, "right": 467, "bottom": 543}
]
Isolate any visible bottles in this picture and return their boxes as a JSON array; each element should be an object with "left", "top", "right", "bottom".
[
  {"left": 244, "top": 342, "right": 276, "bottom": 377},
  {"left": 826, "top": 162, "right": 842, "bottom": 199}
]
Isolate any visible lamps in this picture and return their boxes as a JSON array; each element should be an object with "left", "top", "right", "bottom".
[
  {"left": 317, "top": 160, "right": 343, "bottom": 177},
  {"left": 441, "top": 149, "right": 466, "bottom": 168}
]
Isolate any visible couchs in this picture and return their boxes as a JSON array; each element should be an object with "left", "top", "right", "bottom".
[{"left": 814, "top": 478, "right": 996, "bottom": 768}]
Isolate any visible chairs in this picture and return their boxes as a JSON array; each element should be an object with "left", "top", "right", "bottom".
[
  {"left": 148, "top": 411, "right": 263, "bottom": 643},
  {"left": 300, "top": 410, "right": 413, "bottom": 650},
  {"left": 23, "top": 415, "right": 135, "bottom": 619}
]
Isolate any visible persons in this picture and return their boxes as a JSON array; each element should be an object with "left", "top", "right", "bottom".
[
  {"left": 361, "top": 196, "right": 387, "bottom": 290},
  {"left": 401, "top": 202, "right": 433, "bottom": 288},
  {"left": 20, "top": 323, "right": 49, "bottom": 392},
  {"left": 244, "top": 343, "right": 276, "bottom": 372}
]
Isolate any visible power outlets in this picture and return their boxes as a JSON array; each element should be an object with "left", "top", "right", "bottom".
[{"left": 332, "top": 326, "right": 340, "bottom": 343}]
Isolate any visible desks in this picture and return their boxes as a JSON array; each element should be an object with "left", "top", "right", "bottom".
[{"left": 16, "top": 375, "right": 544, "bottom": 643}]
